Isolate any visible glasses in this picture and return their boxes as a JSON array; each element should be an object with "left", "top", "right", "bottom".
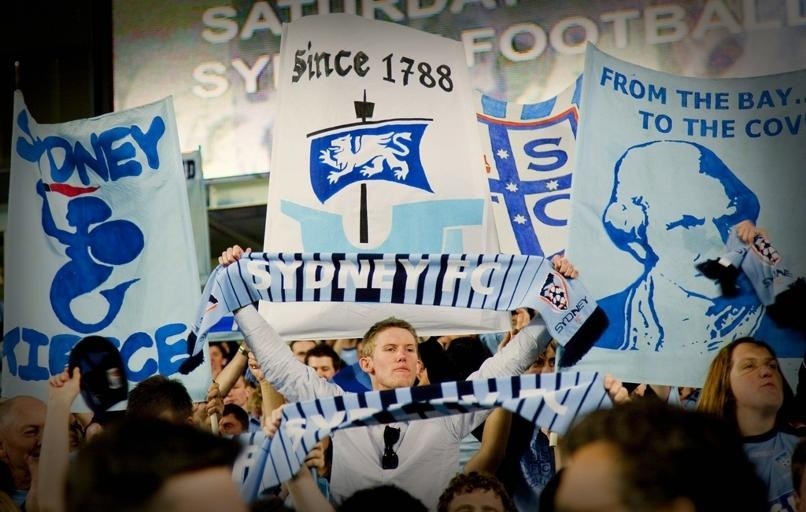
[{"left": 381, "top": 423, "right": 401, "bottom": 471}]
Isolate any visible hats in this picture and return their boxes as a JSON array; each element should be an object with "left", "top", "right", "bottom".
[{"left": 67, "top": 335, "right": 129, "bottom": 413}]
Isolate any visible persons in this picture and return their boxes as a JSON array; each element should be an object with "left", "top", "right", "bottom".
[
  {"left": 585, "top": 139, "right": 804, "bottom": 359},
  {"left": 0, "top": 219, "right": 806, "bottom": 511}
]
